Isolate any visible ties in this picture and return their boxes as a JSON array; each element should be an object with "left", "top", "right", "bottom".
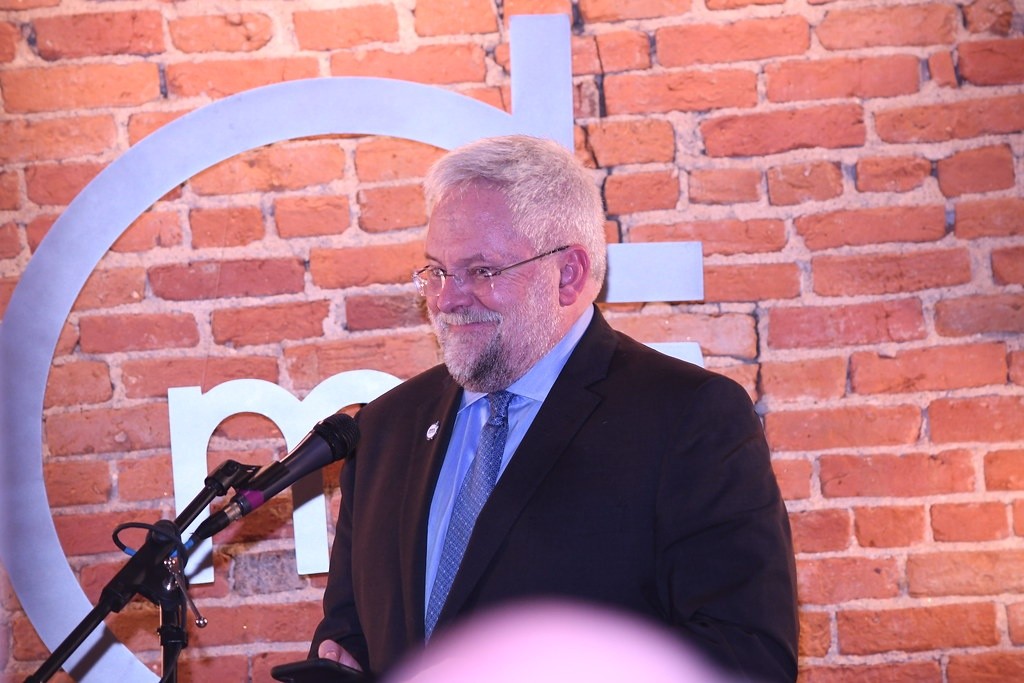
[{"left": 424, "top": 390, "right": 515, "bottom": 649}]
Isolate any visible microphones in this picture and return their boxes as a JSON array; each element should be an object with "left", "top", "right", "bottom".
[{"left": 193, "top": 412, "right": 360, "bottom": 539}]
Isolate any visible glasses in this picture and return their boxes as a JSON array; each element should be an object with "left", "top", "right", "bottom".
[{"left": 412, "top": 245, "right": 570, "bottom": 298}]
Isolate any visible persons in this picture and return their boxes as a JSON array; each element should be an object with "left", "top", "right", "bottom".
[{"left": 305, "top": 134, "right": 800, "bottom": 683}]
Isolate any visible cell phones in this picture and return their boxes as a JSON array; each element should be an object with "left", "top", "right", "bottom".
[{"left": 269, "top": 659, "right": 378, "bottom": 683}]
